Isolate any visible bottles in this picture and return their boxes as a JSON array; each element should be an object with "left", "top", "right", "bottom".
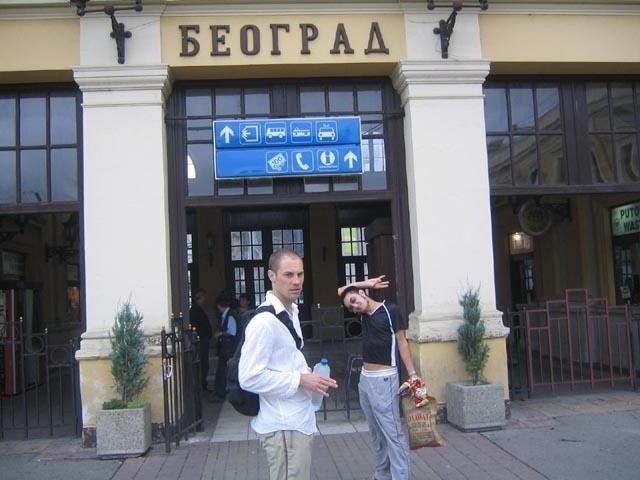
[{"left": 309, "top": 358, "right": 330, "bottom": 412}]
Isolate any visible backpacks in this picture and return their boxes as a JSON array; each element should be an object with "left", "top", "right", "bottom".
[{"left": 224, "top": 305, "right": 303, "bottom": 417}]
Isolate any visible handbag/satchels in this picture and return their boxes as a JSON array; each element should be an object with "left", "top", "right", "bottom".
[{"left": 400, "top": 394, "right": 445, "bottom": 452}]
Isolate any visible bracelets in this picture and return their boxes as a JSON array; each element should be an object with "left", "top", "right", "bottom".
[{"left": 408, "top": 370, "right": 416, "bottom": 377}]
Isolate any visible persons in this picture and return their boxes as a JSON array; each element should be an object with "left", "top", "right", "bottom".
[
  {"left": 224, "top": 249, "right": 338, "bottom": 479},
  {"left": 189, "top": 288, "right": 255, "bottom": 402},
  {"left": 336, "top": 275, "right": 418, "bottom": 480}
]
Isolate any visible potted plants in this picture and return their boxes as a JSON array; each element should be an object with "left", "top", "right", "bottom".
[
  {"left": 94, "top": 292, "right": 153, "bottom": 460},
  {"left": 446, "top": 275, "right": 507, "bottom": 432}
]
